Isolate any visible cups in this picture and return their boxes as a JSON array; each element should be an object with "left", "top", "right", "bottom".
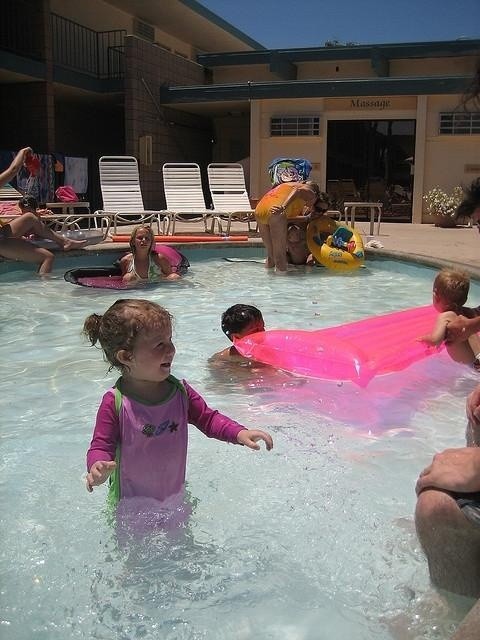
[{"left": 365, "top": 236, "right": 374, "bottom": 243}]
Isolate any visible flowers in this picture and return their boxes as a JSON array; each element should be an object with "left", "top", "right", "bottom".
[{"left": 416, "top": 183, "right": 463, "bottom": 217}]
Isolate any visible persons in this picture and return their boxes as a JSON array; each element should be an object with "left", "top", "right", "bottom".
[
  {"left": 302, "top": 193, "right": 330, "bottom": 225},
  {"left": 414, "top": 267, "right": 480, "bottom": 372},
  {"left": 285, "top": 224, "right": 307, "bottom": 264},
  {"left": 209, "top": 304, "right": 266, "bottom": 367},
  {"left": 119, "top": 223, "right": 181, "bottom": 283},
  {"left": 254, "top": 180, "right": 319, "bottom": 274},
  {"left": 0, "top": 146, "right": 87, "bottom": 274},
  {"left": 80, "top": 298, "right": 274, "bottom": 499},
  {"left": 443, "top": 314, "right": 480, "bottom": 346},
  {"left": 414, "top": 383, "right": 480, "bottom": 605}
]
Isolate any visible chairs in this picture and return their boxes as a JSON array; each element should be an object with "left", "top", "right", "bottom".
[
  {"left": 159, "top": 164, "right": 221, "bottom": 236},
  {"left": 207, "top": 162, "right": 256, "bottom": 236},
  {"left": 326, "top": 177, "right": 361, "bottom": 202},
  {"left": 95, "top": 156, "right": 162, "bottom": 239},
  {"left": 0, "top": 184, "right": 40, "bottom": 221}
]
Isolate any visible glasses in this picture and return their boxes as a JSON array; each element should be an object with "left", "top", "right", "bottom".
[{"left": 314, "top": 204, "right": 328, "bottom": 214}]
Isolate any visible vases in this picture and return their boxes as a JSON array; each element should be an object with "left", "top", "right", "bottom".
[{"left": 434, "top": 214, "right": 453, "bottom": 227}]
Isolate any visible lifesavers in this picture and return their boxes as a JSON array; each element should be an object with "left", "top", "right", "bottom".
[
  {"left": 65, "top": 244, "right": 190, "bottom": 290},
  {"left": 233, "top": 299, "right": 444, "bottom": 388},
  {"left": 305, "top": 215, "right": 366, "bottom": 272}
]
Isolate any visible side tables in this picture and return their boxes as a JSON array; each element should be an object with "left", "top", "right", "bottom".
[
  {"left": 342, "top": 200, "right": 382, "bottom": 236},
  {"left": 46, "top": 202, "right": 92, "bottom": 231}
]
